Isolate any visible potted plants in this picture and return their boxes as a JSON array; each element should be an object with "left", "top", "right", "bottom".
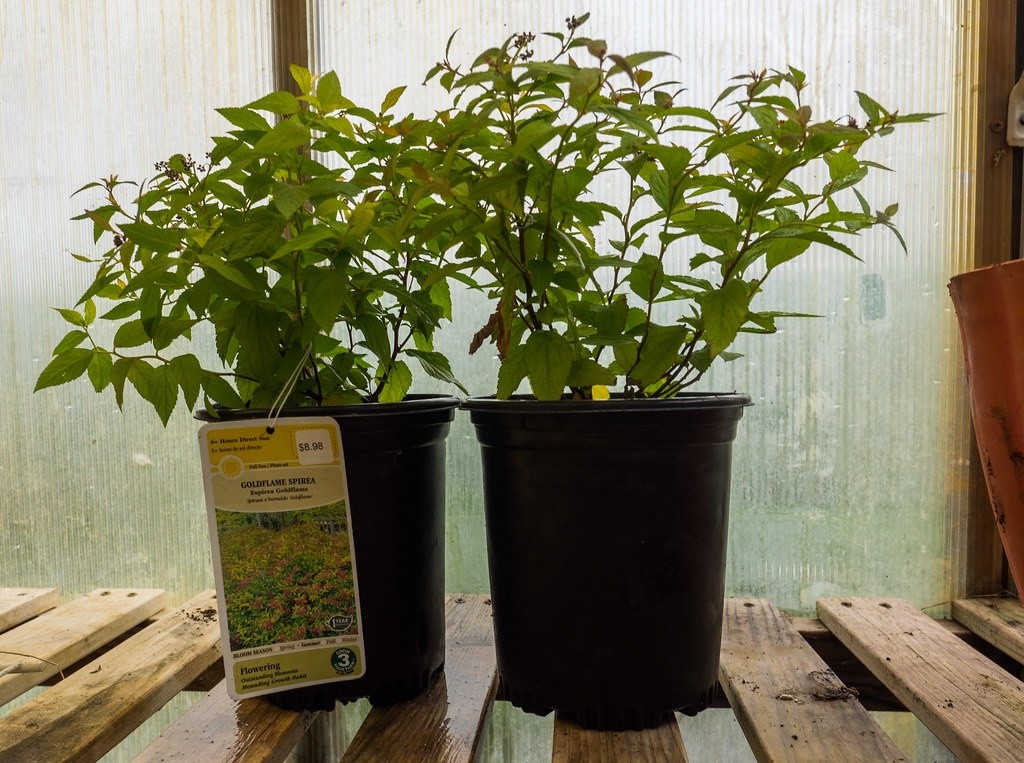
[
  {"left": 33, "top": 62, "right": 460, "bottom": 712},
  {"left": 459, "top": 12, "right": 947, "bottom": 732}
]
